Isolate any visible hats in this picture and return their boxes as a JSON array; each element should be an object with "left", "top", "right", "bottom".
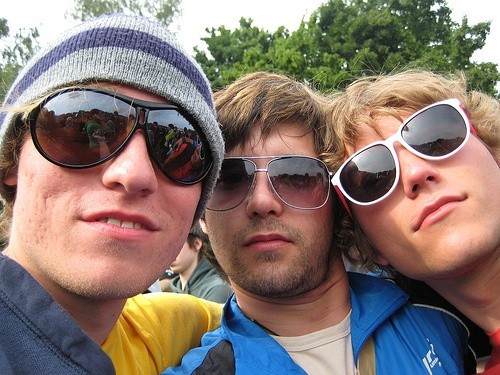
[{"left": 0, "top": 14, "right": 226, "bottom": 233}]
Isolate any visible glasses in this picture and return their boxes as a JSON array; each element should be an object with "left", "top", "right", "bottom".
[
  {"left": 27, "top": 86, "right": 214, "bottom": 186},
  {"left": 206, "top": 154, "right": 335, "bottom": 211},
  {"left": 332, "top": 97, "right": 479, "bottom": 205}
]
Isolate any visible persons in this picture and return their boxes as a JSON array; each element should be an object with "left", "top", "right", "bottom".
[
  {"left": 314, "top": 69, "right": 500, "bottom": 375},
  {"left": 0, "top": 14, "right": 225, "bottom": 375},
  {"left": 101, "top": 223, "right": 232, "bottom": 375},
  {"left": 159, "top": 74, "right": 491, "bottom": 375},
  {"left": 275, "top": 172, "right": 326, "bottom": 206},
  {"left": 148, "top": 121, "right": 206, "bottom": 180},
  {"left": 39, "top": 107, "right": 135, "bottom": 162}
]
